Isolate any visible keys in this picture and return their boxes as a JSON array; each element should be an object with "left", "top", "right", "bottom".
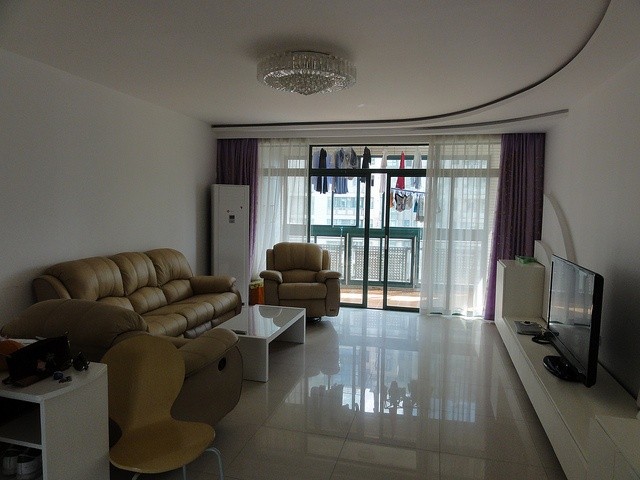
[{"left": 58, "top": 375, "right": 73, "bottom": 384}]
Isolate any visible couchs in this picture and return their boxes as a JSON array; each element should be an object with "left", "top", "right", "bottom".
[
  {"left": 259, "top": 242, "right": 342, "bottom": 318},
  {"left": 5, "top": 299, "right": 243, "bottom": 427},
  {"left": 31, "top": 248, "right": 242, "bottom": 338}
]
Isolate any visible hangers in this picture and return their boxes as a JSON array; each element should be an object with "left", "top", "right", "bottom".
[
  {"left": 387, "top": 186, "right": 426, "bottom": 197},
  {"left": 515, "top": 253, "right": 536, "bottom": 264}
]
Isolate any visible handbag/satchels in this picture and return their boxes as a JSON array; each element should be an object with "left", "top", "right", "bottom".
[{"left": 3, "top": 330, "right": 71, "bottom": 388}]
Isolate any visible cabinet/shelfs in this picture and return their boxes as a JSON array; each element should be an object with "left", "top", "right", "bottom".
[
  {"left": 494, "top": 258, "right": 640, "bottom": 480},
  {"left": 0, "top": 359, "right": 110, "bottom": 480}
]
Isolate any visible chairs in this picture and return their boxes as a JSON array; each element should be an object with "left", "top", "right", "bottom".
[{"left": 99, "top": 335, "right": 224, "bottom": 479}]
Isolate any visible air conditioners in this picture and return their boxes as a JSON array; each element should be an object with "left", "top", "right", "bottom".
[{"left": 210, "top": 183, "right": 249, "bottom": 306}]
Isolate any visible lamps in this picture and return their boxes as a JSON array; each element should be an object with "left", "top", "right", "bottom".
[{"left": 257, "top": 51, "right": 357, "bottom": 97}]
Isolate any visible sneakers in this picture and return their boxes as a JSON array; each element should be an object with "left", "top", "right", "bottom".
[
  {"left": 16, "top": 451, "right": 42, "bottom": 480},
  {"left": 0, "top": 449, "right": 17, "bottom": 475}
]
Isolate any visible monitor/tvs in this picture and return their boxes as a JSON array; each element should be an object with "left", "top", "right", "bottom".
[{"left": 542, "top": 254, "right": 604, "bottom": 388}]
